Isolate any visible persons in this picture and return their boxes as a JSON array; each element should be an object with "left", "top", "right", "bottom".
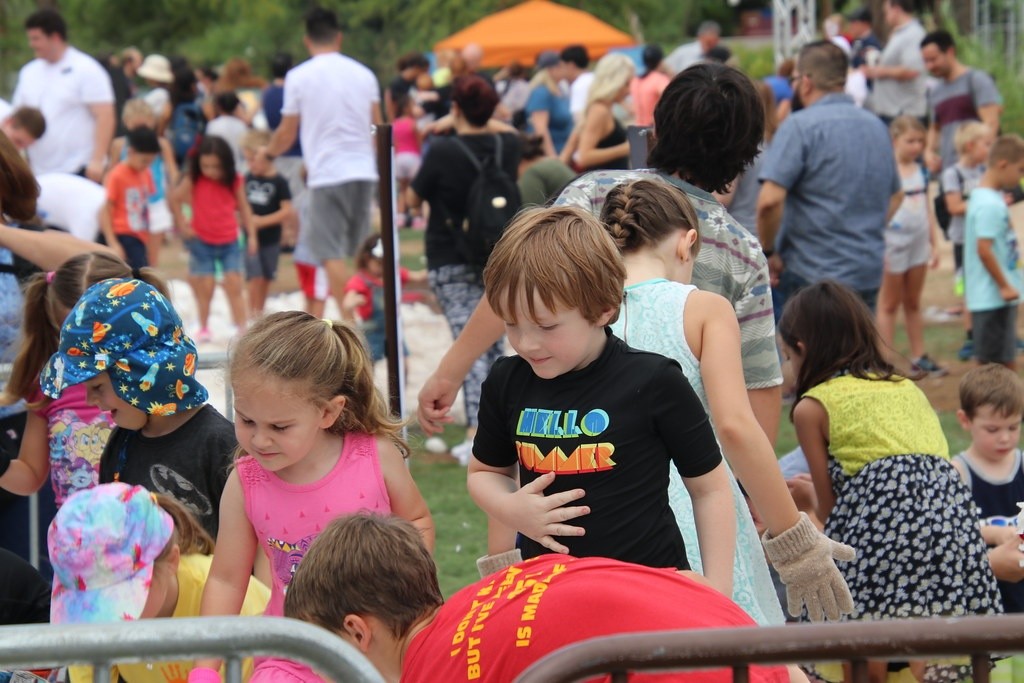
[
  {"left": 949, "top": 364, "right": 1024, "bottom": 614},
  {"left": 384, "top": 11, "right": 880, "bottom": 228},
  {"left": 406, "top": 77, "right": 544, "bottom": 465},
  {"left": 942, "top": 121, "right": 999, "bottom": 274},
  {"left": 465, "top": 205, "right": 736, "bottom": 596},
  {"left": 963, "top": 132, "right": 1023, "bottom": 370},
  {"left": 418, "top": 65, "right": 784, "bottom": 438},
  {"left": 344, "top": 232, "right": 428, "bottom": 369},
  {"left": 250, "top": 8, "right": 382, "bottom": 324},
  {"left": 186, "top": 311, "right": 434, "bottom": 683},
  {"left": 0, "top": 253, "right": 171, "bottom": 512},
  {"left": 779, "top": 281, "right": 1003, "bottom": 683},
  {"left": 858, "top": 1, "right": 943, "bottom": 129},
  {"left": 0, "top": 9, "right": 307, "bottom": 436},
  {"left": 50, "top": 482, "right": 272, "bottom": 683},
  {"left": 57, "top": 278, "right": 239, "bottom": 554},
  {"left": 757, "top": 41, "right": 904, "bottom": 316},
  {"left": 597, "top": 180, "right": 855, "bottom": 683},
  {"left": 877, "top": 116, "right": 948, "bottom": 376},
  {"left": 919, "top": 29, "right": 1003, "bottom": 194},
  {"left": 283, "top": 512, "right": 789, "bottom": 683}
]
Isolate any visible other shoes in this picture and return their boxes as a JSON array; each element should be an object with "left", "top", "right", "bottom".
[
  {"left": 1015, "top": 337, "right": 1024, "bottom": 350},
  {"left": 910, "top": 354, "right": 948, "bottom": 377},
  {"left": 198, "top": 327, "right": 211, "bottom": 342},
  {"left": 958, "top": 338, "right": 977, "bottom": 360},
  {"left": 397, "top": 214, "right": 406, "bottom": 227},
  {"left": 414, "top": 217, "right": 428, "bottom": 227},
  {"left": 451, "top": 438, "right": 476, "bottom": 458}
]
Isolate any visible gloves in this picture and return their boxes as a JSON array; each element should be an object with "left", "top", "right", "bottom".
[{"left": 761, "top": 511, "right": 857, "bottom": 624}]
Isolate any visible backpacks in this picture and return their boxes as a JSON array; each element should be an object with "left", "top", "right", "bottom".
[
  {"left": 452, "top": 132, "right": 519, "bottom": 251},
  {"left": 934, "top": 166, "right": 965, "bottom": 239}
]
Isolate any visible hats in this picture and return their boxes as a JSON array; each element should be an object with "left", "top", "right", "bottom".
[
  {"left": 40, "top": 278, "right": 209, "bottom": 415},
  {"left": 135, "top": 54, "right": 176, "bottom": 84},
  {"left": 47, "top": 482, "right": 173, "bottom": 625}
]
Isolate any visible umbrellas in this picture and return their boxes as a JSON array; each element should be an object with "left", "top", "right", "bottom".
[{"left": 433, "top": 0, "right": 635, "bottom": 64}]
